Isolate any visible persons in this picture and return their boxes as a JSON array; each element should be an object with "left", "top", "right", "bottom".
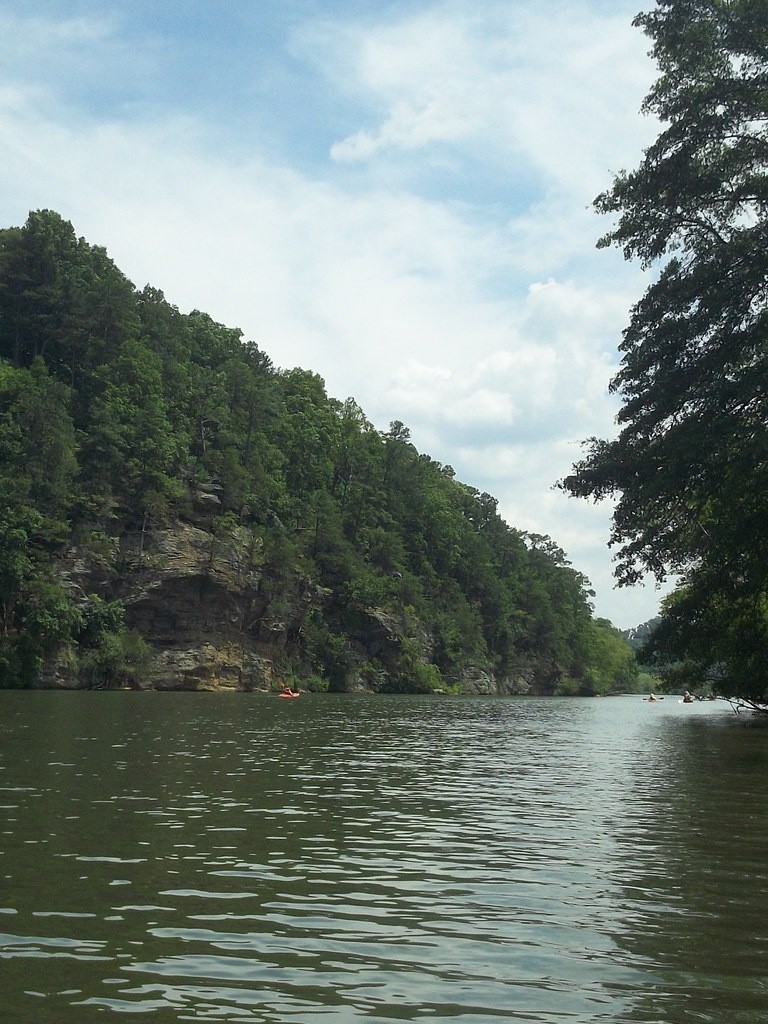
[{"left": 283, "top": 686, "right": 292, "bottom": 694}]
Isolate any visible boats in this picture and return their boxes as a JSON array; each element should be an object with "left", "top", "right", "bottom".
[
  {"left": 648, "top": 696, "right": 656, "bottom": 702},
  {"left": 278, "top": 692, "right": 300, "bottom": 698},
  {"left": 683, "top": 699, "right": 694, "bottom": 703}
]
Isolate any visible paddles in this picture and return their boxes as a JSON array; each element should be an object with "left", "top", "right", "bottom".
[{"left": 642, "top": 696, "right": 665, "bottom": 702}]
